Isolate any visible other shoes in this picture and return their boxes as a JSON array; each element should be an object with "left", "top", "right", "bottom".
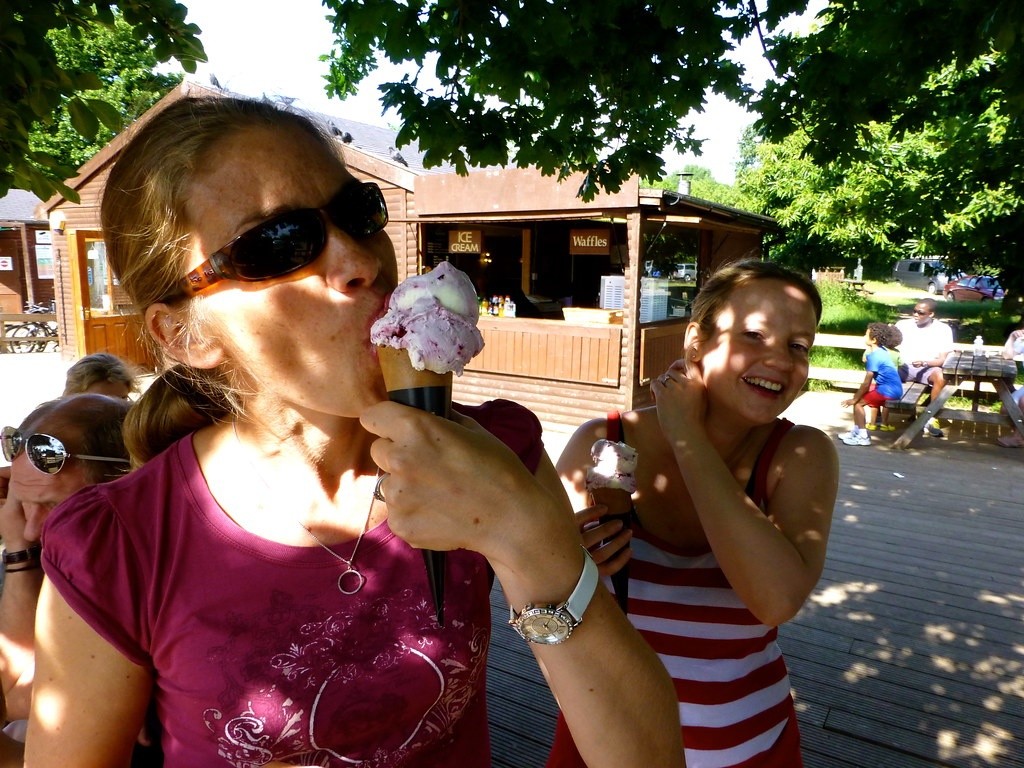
[
  {"left": 881, "top": 424, "right": 895, "bottom": 431},
  {"left": 865, "top": 423, "right": 877, "bottom": 431}
]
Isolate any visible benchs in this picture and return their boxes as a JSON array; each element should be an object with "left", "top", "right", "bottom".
[{"left": 884, "top": 381, "right": 928, "bottom": 415}]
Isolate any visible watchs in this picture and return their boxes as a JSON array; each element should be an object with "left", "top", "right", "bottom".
[{"left": 508, "top": 544, "right": 599, "bottom": 645}]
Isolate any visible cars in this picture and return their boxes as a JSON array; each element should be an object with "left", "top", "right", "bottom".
[{"left": 942, "top": 274, "right": 1005, "bottom": 303}]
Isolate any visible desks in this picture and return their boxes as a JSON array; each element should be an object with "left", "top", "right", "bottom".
[{"left": 890, "top": 344, "right": 1024, "bottom": 450}]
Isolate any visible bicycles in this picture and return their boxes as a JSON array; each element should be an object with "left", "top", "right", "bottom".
[{"left": 3, "top": 301, "right": 59, "bottom": 353}]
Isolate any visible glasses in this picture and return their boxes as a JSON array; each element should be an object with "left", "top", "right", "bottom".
[
  {"left": 159, "top": 182, "right": 390, "bottom": 306},
  {"left": 0, "top": 426, "right": 137, "bottom": 474},
  {"left": 912, "top": 308, "right": 929, "bottom": 315}
]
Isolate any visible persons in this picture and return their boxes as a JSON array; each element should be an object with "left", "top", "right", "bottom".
[
  {"left": 0, "top": 352, "right": 242, "bottom": 768},
  {"left": 23, "top": 94, "right": 688, "bottom": 768},
  {"left": 837, "top": 323, "right": 903, "bottom": 446},
  {"left": 996, "top": 330, "right": 1024, "bottom": 447},
  {"left": 545, "top": 259, "right": 840, "bottom": 768},
  {"left": 865, "top": 298, "right": 953, "bottom": 436}
]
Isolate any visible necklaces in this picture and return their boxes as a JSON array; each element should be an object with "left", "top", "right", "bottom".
[{"left": 230, "top": 414, "right": 381, "bottom": 595}]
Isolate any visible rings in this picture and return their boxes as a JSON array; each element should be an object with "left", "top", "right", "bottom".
[
  {"left": 664, "top": 376, "right": 669, "bottom": 383},
  {"left": 373, "top": 473, "right": 389, "bottom": 502}
]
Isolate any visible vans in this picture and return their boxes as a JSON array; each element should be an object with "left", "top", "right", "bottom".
[
  {"left": 892, "top": 258, "right": 966, "bottom": 295},
  {"left": 668, "top": 263, "right": 695, "bottom": 282}
]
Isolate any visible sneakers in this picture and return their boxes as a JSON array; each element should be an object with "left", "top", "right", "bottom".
[
  {"left": 838, "top": 428, "right": 859, "bottom": 440},
  {"left": 923, "top": 417, "right": 945, "bottom": 437},
  {"left": 997, "top": 429, "right": 1024, "bottom": 447},
  {"left": 845, "top": 435, "right": 871, "bottom": 445}
]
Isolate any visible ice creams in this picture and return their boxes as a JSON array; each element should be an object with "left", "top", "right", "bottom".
[
  {"left": 369, "top": 260, "right": 486, "bottom": 377},
  {"left": 587, "top": 438, "right": 638, "bottom": 495}
]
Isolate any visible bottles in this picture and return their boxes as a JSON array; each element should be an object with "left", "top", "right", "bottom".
[
  {"left": 973, "top": 336, "right": 984, "bottom": 356},
  {"left": 477, "top": 295, "right": 517, "bottom": 318}
]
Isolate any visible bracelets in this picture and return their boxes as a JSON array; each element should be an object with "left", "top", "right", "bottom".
[
  {"left": 2, "top": 547, "right": 40, "bottom": 565},
  {"left": 923, "top": 361, "right": 928, "bottom": 367},
  {"left": 5, "top": 564, "right": 38, "bottom": 573}
]
